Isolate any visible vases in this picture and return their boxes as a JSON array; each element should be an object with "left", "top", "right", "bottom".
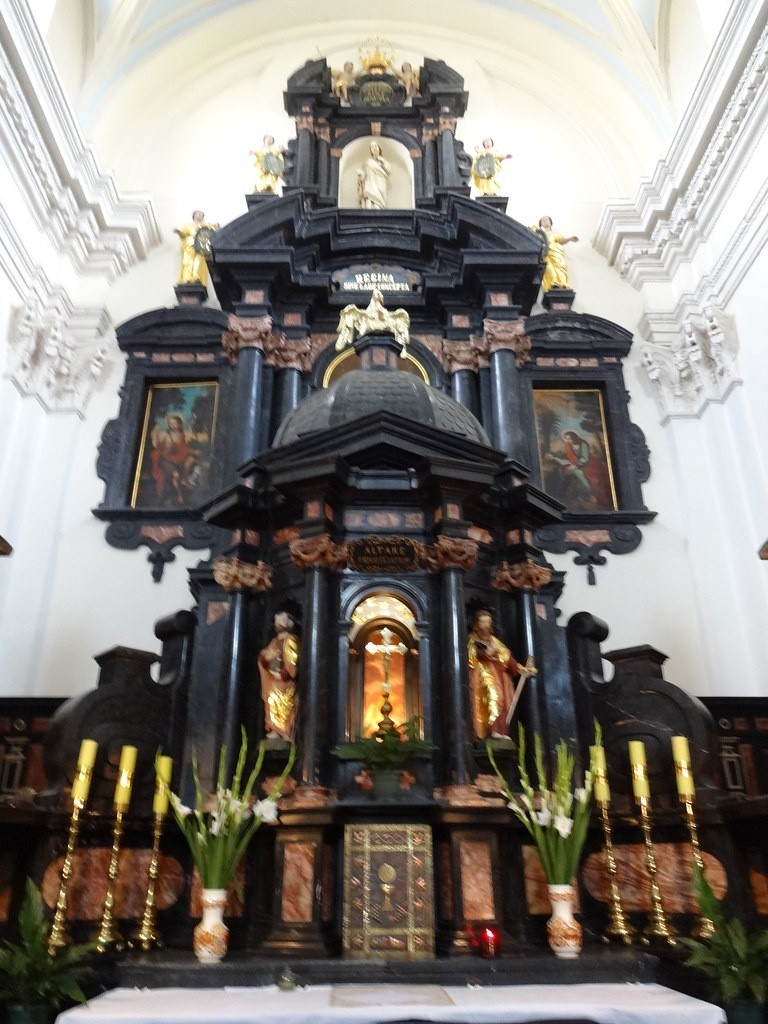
[
  {"left": 546, "top": 884, "right": 582, "bottom": 960},
  {"left": 194, "top": 889, "right": 230, "bottom": 963}
]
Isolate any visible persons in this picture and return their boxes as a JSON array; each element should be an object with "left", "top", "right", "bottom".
[
  {"left": 330, "top": 59, "right": 360, "bottom": 102},
  {"left": 528, "top": 215, "right": 580, "bottom": 291},
  {"left": 389, "top": 61, "right": 423, "bottom": 97},
  {"left": 356, "top": 139, "right": 391, "bottom": 210},
  {"left": 254, "top": 133, "right": 285, "bottom": 191},
  {"left": 468, "top": 610, "right": 536, "bottom": 735},
  {"left": 473, "top": 138, "right": 513, "bottom": 196},
  {"left": 173, "top": 209, "right": 215, "bottom": 284},
  {"left": 257, "top": 612, "right": 297, "bottom": 743}
]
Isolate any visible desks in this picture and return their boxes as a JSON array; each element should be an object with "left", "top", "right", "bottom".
[
  {"left": 56, "top": 983, "right": 729, "bottom": 1024},
  {"left": 117, "top": 948, "right": 659, "bottom": 989}
]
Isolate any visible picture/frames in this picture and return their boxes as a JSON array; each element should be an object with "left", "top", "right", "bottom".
[
  {"left": 130, "top": 382, "right": 219, "bottom": 509},
  {"left": 533, "top": 389, "right": 618, "bottom": 513}
]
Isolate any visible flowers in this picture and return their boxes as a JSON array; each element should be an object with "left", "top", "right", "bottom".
[
  {"left": 486, "top": 718, "right": 602, "bottom": 885},
  {"left": 155, "top": 724, "right": 297, "bottom": 889}
]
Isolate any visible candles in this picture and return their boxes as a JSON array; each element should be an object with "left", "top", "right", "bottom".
[
  {"left": 630, "top": 742, "right": 652, "bottom": 805},
  {"left": 671, "top": 736, "right": 699, "bottom": 803},
  {"left": 153, "top": 756, "right": 174, "bottom": 813},
  {"left": 590, "top": 744, "right": 611, "bottom": 802},
  {"left": 113, "top": 746, "right": 138, "bottom": 812},
  {"left": 70, "top": 740, "right": 99, "bottom": 801}
]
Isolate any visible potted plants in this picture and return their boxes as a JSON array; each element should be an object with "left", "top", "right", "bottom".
[
  {"left": 0, "top": 875, "right": 108, "bottom": 1024},
  {"left": 675, "top": 862, "right": 768, "bottom": 1024},
  {"left": 329, "top": 713, "right": 438, "bottom": 798}
]
